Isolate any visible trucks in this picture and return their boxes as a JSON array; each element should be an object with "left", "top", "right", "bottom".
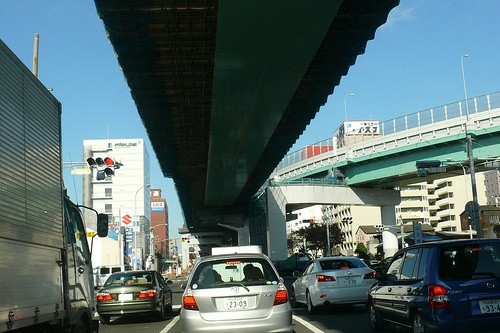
[
  {"left": 0, "top": 38, "right": 110, "bottom": 333},
  {"left": 275, "top": 253, "right": 314, "bottom": 279}
]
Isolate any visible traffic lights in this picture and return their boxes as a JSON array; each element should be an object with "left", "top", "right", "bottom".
[
  {"left": 87, "top": 157, "right": 113, "bottom": 166},
  {"left": 104, "top": 168, "right": 112, "bottom": 175},
  {"left": 343, "top": 217, "right": 353, "bottom": 224},
  {"left": 375, "top": 224, "right": 384, "bottom": 231},
  {"left": 303, "top": 219, "right": 314, "bottom": 223}
]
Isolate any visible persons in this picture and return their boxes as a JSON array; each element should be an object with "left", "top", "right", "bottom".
[{"left": 241, "top": 264, "right": 254, "bottom": 282}]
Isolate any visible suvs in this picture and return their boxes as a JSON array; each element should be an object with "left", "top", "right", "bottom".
[{"left": 367, "top": 238, "right": 500, "bottom": 333}]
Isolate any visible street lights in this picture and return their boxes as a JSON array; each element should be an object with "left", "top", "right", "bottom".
[
  {"left": 344, "top": 93, "right": 354, "bottom": 120},
  {"left": 134, "top": 184, "right": 151, "bottom": 269},
  {"left": 460, "top": 54, "right": 469, "bottom": 115},
  {"left": 150, "top": 223, "right": 167, "bottom": 254}
]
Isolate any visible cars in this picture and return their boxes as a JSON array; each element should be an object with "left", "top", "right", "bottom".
[
  {"left": 95, "top": 268, "right": 174, "bottom": 324},
  {"left": 290, "top": 256, "right": 380, "bottom": 314},
  {"left": 181, "top": 251, "right": 295, "bottom": 332}
]
